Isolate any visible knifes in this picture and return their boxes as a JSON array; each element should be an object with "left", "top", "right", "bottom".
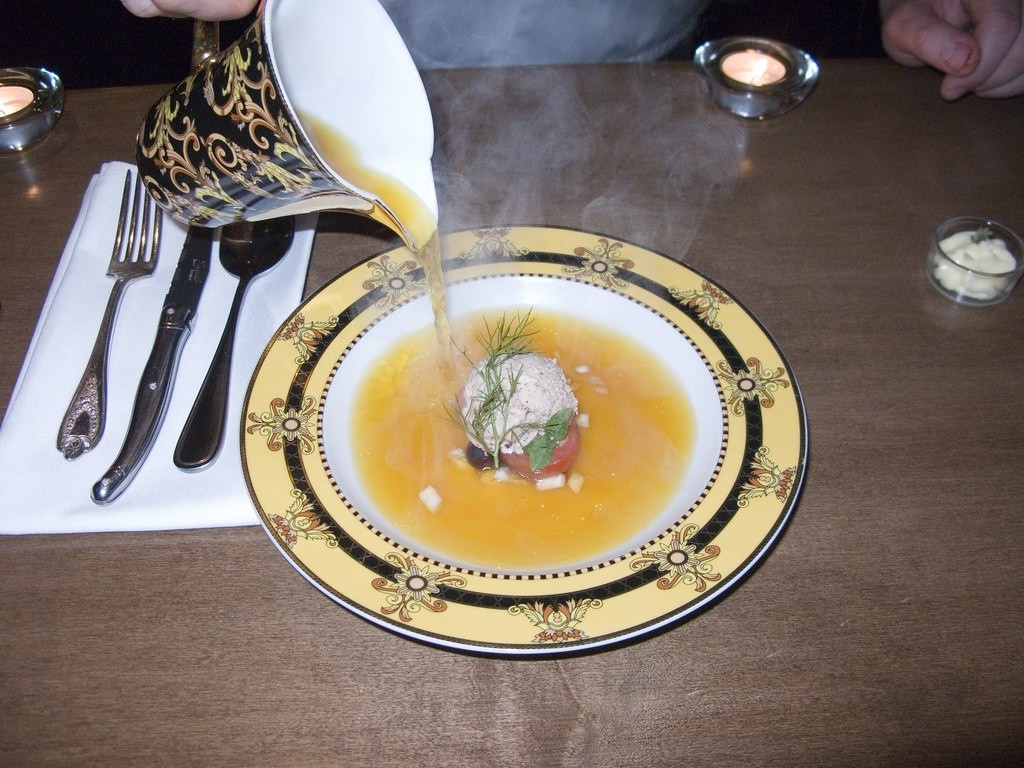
[{"left": 88, "top": 225, "right": 213, "bottom": 504}]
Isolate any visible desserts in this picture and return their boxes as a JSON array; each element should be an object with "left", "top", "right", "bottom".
[{"left": 464, "top": 353, "right": 582, "bottom": 476}]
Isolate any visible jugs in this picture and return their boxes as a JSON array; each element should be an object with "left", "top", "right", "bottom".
[{"left": 135, "top": 0, "right": 438, "bottom": 253}]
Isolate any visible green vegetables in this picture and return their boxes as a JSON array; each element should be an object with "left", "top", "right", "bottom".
[{"left": 437, "top": 305, "right": 575, "bottom": 473}]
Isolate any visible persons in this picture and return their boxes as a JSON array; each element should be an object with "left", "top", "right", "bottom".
[{"left": 120, "top": 0, "right": 1024, "bottom": 101}]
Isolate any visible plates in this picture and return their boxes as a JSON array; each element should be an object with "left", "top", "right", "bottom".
[{"left": 241, "top": 224, "right": 811, "bottom": 660}]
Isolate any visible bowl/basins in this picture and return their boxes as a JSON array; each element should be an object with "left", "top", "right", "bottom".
[
  {"left": 0, "top": 66, "right": 64, "bottom": 152},
  {"left": 695, "top": 35, "right": 818, "bottom": 119},
  {"left": 927, "top": 215, "right": 1024, "bottom": 307}
]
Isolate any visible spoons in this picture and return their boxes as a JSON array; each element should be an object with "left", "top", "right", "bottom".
[{"left": 173, "top": 215, "right": 296, "bottom": 473}]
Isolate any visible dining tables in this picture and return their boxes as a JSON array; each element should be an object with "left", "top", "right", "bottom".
[{"left": 0, "top": 58, "right": 1024, "bottom": 768}]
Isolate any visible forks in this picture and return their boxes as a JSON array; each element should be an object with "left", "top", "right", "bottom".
[{"left": 57, "top": 167, "right": 163, "bottom": 461}]
